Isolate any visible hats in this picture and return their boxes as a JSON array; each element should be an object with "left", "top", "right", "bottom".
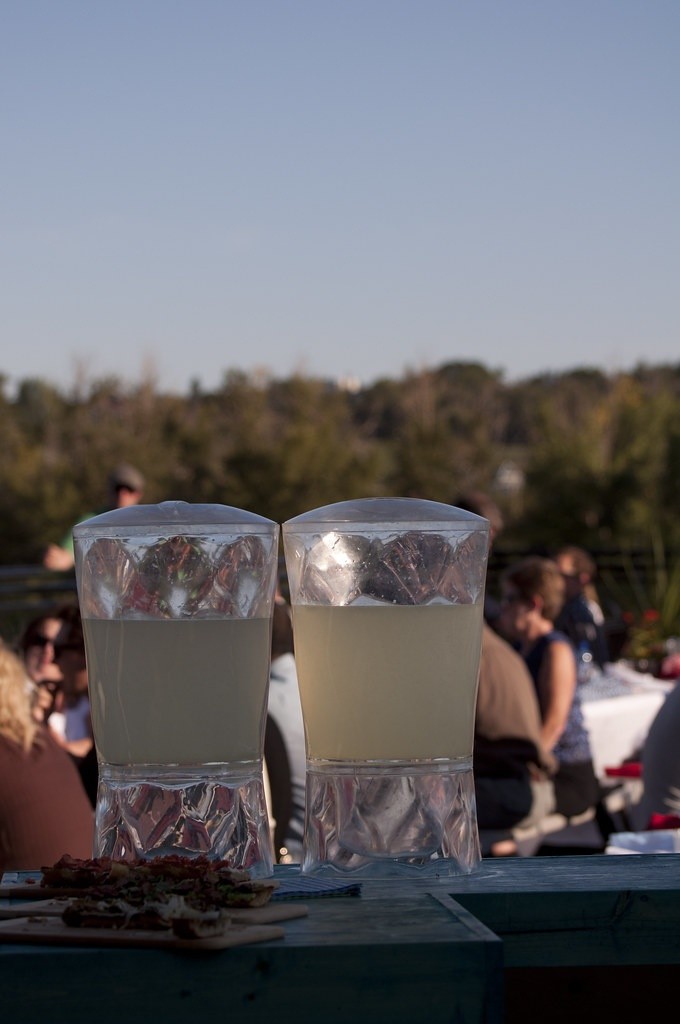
[{"left": 108, "top": 464, "right": 144, "bottom": 492}]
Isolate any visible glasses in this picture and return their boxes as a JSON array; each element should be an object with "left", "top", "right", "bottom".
[{"left": 23, "top": 627, "right": 64, "bottom": 656}]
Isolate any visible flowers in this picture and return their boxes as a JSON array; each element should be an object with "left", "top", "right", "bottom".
[{"left": 620, "top": 556, "right": 680, "bottom": 680}]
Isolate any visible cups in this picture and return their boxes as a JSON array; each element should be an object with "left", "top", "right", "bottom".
[
  {"left": 73, "top": 499, "right": 281, "bottom": 882},
  {"left": 279, "top": 497, "right": 492, "bottom": 879}
]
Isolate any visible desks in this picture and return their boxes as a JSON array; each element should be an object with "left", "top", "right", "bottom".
[
  {"left": 0, "top": 854, "right": 680, "bottom": 1024},
  {"left": 549, "top": 675, "right": 677, "bottom": 847}
]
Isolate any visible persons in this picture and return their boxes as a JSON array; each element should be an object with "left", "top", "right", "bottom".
[{"left": 0, "top": 464, "right": 680, "bottom": 874}]
